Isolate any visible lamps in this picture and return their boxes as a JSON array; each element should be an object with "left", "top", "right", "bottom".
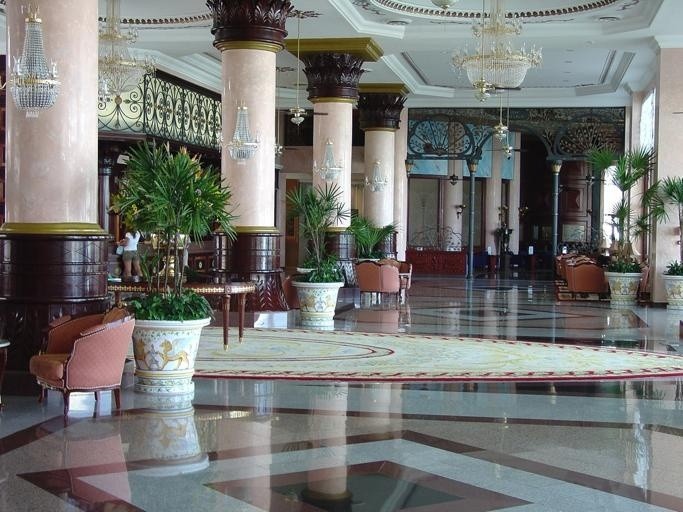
[
  {"left": 364, "top": 159, "right": 389, "bottom": 194},
  {"left": 314, "top": 138, "right": 342, "bottom": 183},
  {"left": 502, "top": 88, "right": 515, "bottom": 161},
  {"left": 404, "top": 154, "right": 414, "bottom": 175},
  {"left": 495, "top": 93, "right": 506, "bottom": 143},
  {"left": 519, "top": 198, "right": 529, "bottom": 218},
  {"left": 451, "top": 0, "right": 542, "bottom": 95},
  {"left": 226, "top": 102, "right": 258, "bottom": 161},
  {"left": 449, "top": 117, "right": 459, "bottom": 184},
  {"left": 289, "top": 10, "right": 318, "bottom": 127},
  {"left": 274, "top": 68, "right": 285, "bottom": 157},
  {"left": 457, "top": 204, "right": 466, "bottom": 216},
  {"left": 2, "top": 3, "right": 62, "bottom": 119},
  {"left": 473, "top": 0, "right": 489, "bottom": 103},
  {"left": 96, "top": 0, "right": 157, "bottom": 102}
]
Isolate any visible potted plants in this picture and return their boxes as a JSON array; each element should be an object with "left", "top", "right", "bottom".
[
  {"left": 589, "top": 148, "right": 655, "bottom": 310},
  {"left": 279, "top": 184, "right": 349, "bottom": 331},
  {"left": 657, "top": 174, "right": 683, "bottom": 310},
  {"left": 109, "top": 140, "right": 239, "bottom": 416},
  {"left": 346, "top": 214, "right": 398, "bottom": 263}
]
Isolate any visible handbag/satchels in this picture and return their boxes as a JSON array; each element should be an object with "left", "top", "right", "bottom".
[{"left": 116, "top": 245, "right": 123, "bottom": 254}]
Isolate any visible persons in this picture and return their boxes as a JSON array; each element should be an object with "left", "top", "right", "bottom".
[{"left": 122, "top": 225, "right": 143, "bottom": 277}]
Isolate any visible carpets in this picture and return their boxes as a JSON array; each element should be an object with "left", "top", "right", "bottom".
[
  {"left": 555, "top": 278, "right": 611, "bottom": 301},
  {"left": 192, "top": 327, "right": 683, "bottom": 376}
]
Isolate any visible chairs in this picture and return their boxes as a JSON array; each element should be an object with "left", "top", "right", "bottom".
[
  {"left": 354, "top": 261, "right": 401, "bottom": 310},
  {"left": 29, "top": 308, "right": 135, "bottom": 427},
  {"left": 378, "top": 257, "right": 413, "bottom": 304},
  {"left": 554, "top": 252, "right": 609, "bottom": 297}
]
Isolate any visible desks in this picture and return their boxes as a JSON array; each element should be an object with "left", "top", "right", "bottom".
[{"left": 107, "top": 280, "right": 255, "bottom": 351}]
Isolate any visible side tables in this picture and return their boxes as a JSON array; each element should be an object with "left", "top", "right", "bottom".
[{"left": 399, "top": 272, "right": 410, "bottom": 305}]
